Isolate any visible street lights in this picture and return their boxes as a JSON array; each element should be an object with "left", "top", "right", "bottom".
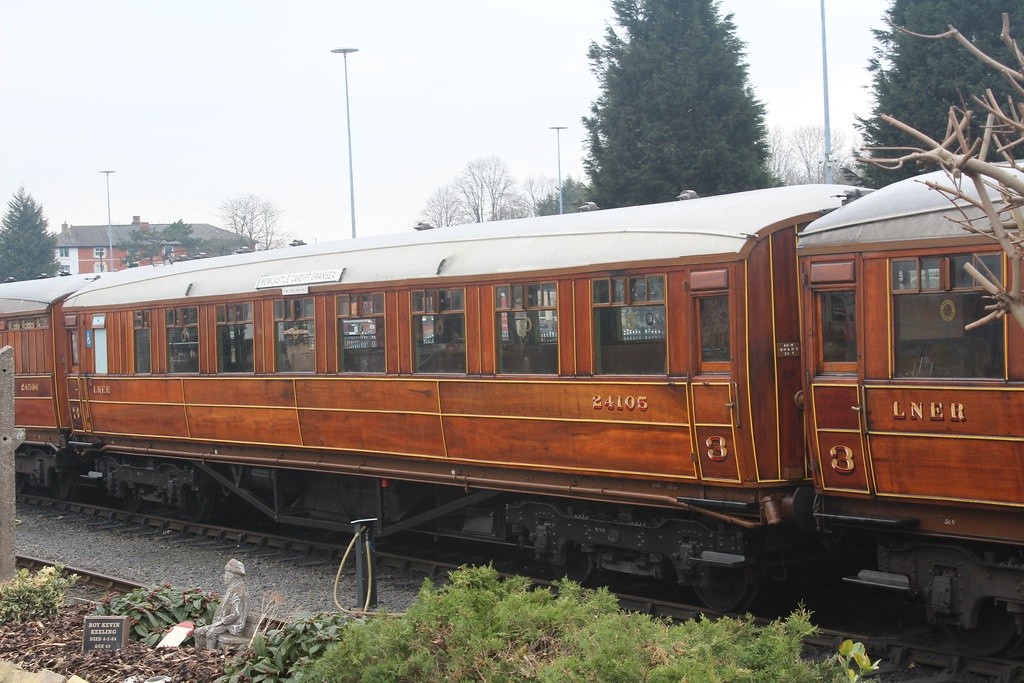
[
  {"left": 548, "top": 127, "right": 568, "bottom": 214},
  {"left": 331, "top": 48, "right": 358, "bottom": 238},
  {"left": 99, "top": 171, "right": 117, "bottom": 272}
]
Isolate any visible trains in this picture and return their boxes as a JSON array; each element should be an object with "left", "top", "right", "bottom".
[{"left": 0, "top": 162, "right": 1023, "bottom": 659}]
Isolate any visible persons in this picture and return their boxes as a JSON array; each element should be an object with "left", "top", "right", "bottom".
[{"left": 193, "top": 559, "right": 250, "bottom": 651}]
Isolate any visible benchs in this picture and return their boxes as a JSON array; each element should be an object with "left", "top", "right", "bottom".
[
  {"left": 501, "top": 340, "right": 557, "bottom": 374},
  {"left": 601, "top": 336, "right": 665, "bottom": 374},
  {"left": 345, "top": 348, "right": 383, "bottom": 373},
  {"left": 420, "top": 342, "right": 466, "bottom": 371}
]
